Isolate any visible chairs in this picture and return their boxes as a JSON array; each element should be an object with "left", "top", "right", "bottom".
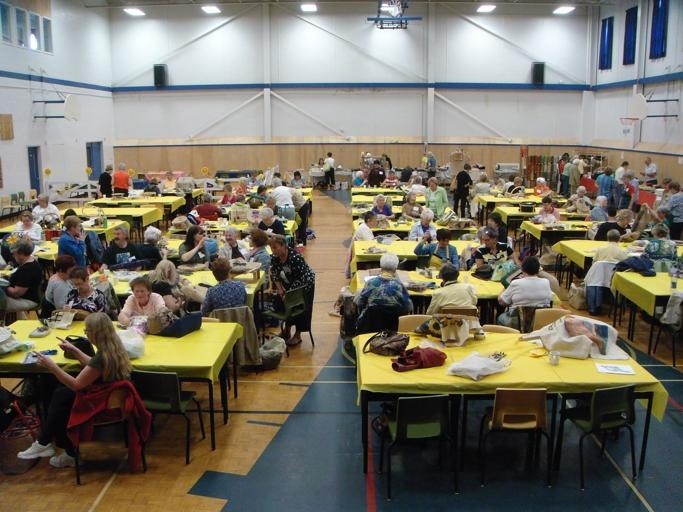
[
  {"left": 3, "top": 278, "right": 44, "bottom": 325},
  {"left": 10, "top": 192, "right": 30, "bottom": 216},
  {"left": 532, "top": 307, "right": 572, "bottom": 332},
  {"left": 653, "top": 289, "right": 683, "bottom": 367},
  {"left": 478, "top": 387, "right": 554, "bottom": 488},
  {"left": 261, "top": 284, "right": 314, "bottom": 358},
  {"left": 17, "top": 191, "right": 32, "bottom": 211},
  {"left": 482, "top": 323, "right": 521, "bottom": 335},
  {"left": 396, "top": 314, "right": 433, "bottom": 332},
  {"left": 1, "top": 194, "right": 21, "bottom": 221},
  {"left": 65, "top": 379, "right": 152, "bottom": 484},
  {"left": 29, "top": 188, "right": 39, "bottom": 207},
  {"left": 378, "top": 393, "right": 460, "bottom": 502},
  {"left": 441, "top": 305, "right": 478, "bottom": 317},
  {"left": 131, "top": 369, "right": 205, "bottom": 472},
  {"left": 555, "top": 384, "right": 637, "bottom": 491},
  {"left": 514, "top": 215, "right": 535, "bottom": 251},
  {"left": 115, "top": 214, "right": 138, "bottom": 244}
]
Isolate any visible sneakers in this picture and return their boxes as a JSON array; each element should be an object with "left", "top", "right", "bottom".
[
  {"left": 49, "top": 449, "right": 81, "bottom": 468},
  {"left": 16, "top": 439, "right": 56, "bottom": 460}
]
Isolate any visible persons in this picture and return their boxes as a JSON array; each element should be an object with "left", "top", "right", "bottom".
[
  {"left": 487, "top": 212, "right": 509, "bottom": 243},
  {"left": 453, "top": 163, "right": 474, "bottom": 218},
  {"left": 118, "top": 276, "right": 166, "bottom": 326},
  {"left": 248, "top": 228, "right": 271, "bottom": 291},
  {"left": 538, "top": 196, "right": 560, "bottom": 222},
  {"left": 352, "top": 251, "right": 414, "bottom": 335},
  {"left": 408, "top": 208, "right": 438, "bottom": 241},
  {"left": 291, "top": 170, "right": 306, "bottom": 188},
  {"left": 291, "top": 189, "right": 311, "bottom": 243},
  {"left": 352, "top": 153, "right": 427, "bottom": 195},
  {"left": 105, "top": 225, "right": 142, "bottom": 271},
  {"left": 594, "top": 208, "right": 641, "bottom": 242},
  {"left": 201, "top": 257, "right": 248, "bottom": 317},
  {"left": 194, "top": 171, "right": 296, "bottom": 236},
  {"left": 426, "top": 151, "right": 437, "bottom": 178},
  {"left": 323, "top": 152, "right": 337, "bottom": 191},
  {"left": 178, "top": 225, "right": 209, "bottom": 264},
  {"left": 508, "top": 176, "right": 525, "bottom": 194},
  {"left": 151, "top": 258, "right": 186, "bottom": 317},
  {"left": 592, "top": 228, "right": 628, "bottom": 267},
  {"left": 111, "top": 164, "right": 133, "bottom": 197},
  {"left": 44, "top": 255, "right": 77, "bottom": 310},
  {"left": 161, "top": 172, "right": 177, "bottom": 192},
  {"left": 32, "top": 193, "right": 61, "bottom": 225},
  {"left": 17, "top": 312, "right": 133, "bottom": 468},
  {"left": 12, "top": 210, "right": 43, "bottom": 240},
  {"left": 62, "top": 267, "right": 107, "bottom": 319},
  {"left": 640, "top": 223, "right": 678, "bottom": 259},
  {"left": 502, "top": 174, "right": 515, "bottom": 194},
  {"left": 143, "top": 177, "right": 162, "bottom": 198},
  {"left": 267, "top": 234, "right": 316, "bottom": 346},
  {"left": 463, "top": 225, "right": 487, "bottom": 270},
  {"left": 0, "top": 239, "right": 44, "bottom": 312},
  {"left": 474, "top": 227, "right": 515, "bottom": 268},
  {"left": 98, "top": 164, "right": 113, "bottom": 198},
  {"left": 639, "top": 156, "right": 683, "bottom": 227},
  {"left": 218, "top": 225, "right": 251, "bottom": 262},
  {"left": 75, "top": 224, "right": 104, "bottom": 274},
  {"left": 556, "top": 151, "right": 639, "bottom": 220},
  {"left": 401, "top": 191, "right": 424, "bottom": 220},
  {"left": 57, "top": 215, "right": 88, "bottom": 269},
  {"left": 350, "top": 210, "right": 378, "bottom": 242},
  {"left": 426, "top": 263, "right": 479, "bottom": 315},
  {"left": 534, "top": 176, "right": 550, "bottom": 196},
  {"left": 372, "top": 193, "right": 396, "bottom": 220},
  {"left": 414, "top": 228, "right": 461, "bottom": 273},
  {"left": 424, "top": 176, "right": 449, "bottom": 217},
  {"left": 498, "top": 255, "right": 551, "bottom": 327},
  {"left": 475, "top": 171, "right": 491, "bottom": 194},
  {"left": 317, "top": 158, "right": 325, "bottom": 169},
  {"left": 139, "top": 225, "right": 169, "bottom": 270}
]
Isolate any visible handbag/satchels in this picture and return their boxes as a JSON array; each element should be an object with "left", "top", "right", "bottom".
[
  {"left": 64, "top": 334, "right": 96, "bottom": 361},
  {"left": 146, "top": 306, "right": 173, "bottom": 335},
  {"left": 567, "top": 281, "right": 588, "bottom": 310},
  {"left": 362, "top": 328, "right": 411, "bottom": 357}
]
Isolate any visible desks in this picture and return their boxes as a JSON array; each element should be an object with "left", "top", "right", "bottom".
[
  {"left": 162, "top": 188, "right": 204, "bottom": 209},
  {"left": 309, "top": 166, "right": 480, "bottom": 191},
  {"left": 466, "top": 187, "right": 535, "bottom": 216},
  {"left": 246, "top": 186, "right": 314, "bottom": 198},
  {"left": 610, "top": 269, "right": 683, "bottom": 355},
  {"left": 352, "top": 216, "right": 478, "bottom": 240},
  {"left": 138, "top": 170, "right": 183, "bottom": 180},
  {"left": 88, "top": 196, "right": 187, "bottom": 229},
  {"left": 215, "top": 169, "right": 262, "bottom": 178},
  {"left": 493, "top": 206, "right": 592, "bottom": 243},
  {"left": 478, "top": 195, "right": 568, "bottom": 227},
  {"left": 348, "top": 268, "right": 506, "bottom": 327},
  {"left": 349, "top": 240, "right": 479, "bottom": 278},
  {"left": 194, "top": 177, "right": 253, "bottom": 186},
  {"left": 213, "top": 202, "right": 266, "bottom": 216},
  {"left": 350, "top": 186, "right": 402, "bottom": 196},
  {"left": 551, "top": 238, "right": 683, "bottom": 290},
  {"left": 519, "top": 220, "right": 605, "bottom": 257},
  {"left": 0, "top": 319, "right": 243, "bottom": 450},
  {"left": 132, "top": 178, "right": 149, "bottom": 190},
  {"left": 580, "top": 174, "right": 663, "bottom": 210},
  {"left": 88, "top": 268, "right": 267, "bottom": 345},
  {"left": 351, "top": 194, "right": 426, "bottom": 206},
  {"left": 351, "top": 204, "right": 455, "bottom": 220},
  {"left": 303, "top": 195, "right": 311, "bottom": 226},
  {"left": 58, "top": 207, "right": 163, "bottom": 245},
  {"left": 169, "top": 220, "right": 299, "bottom": 249},
  {"left": 157, "top": 238, "right": 273, "bottom": 259},
  {"left": 0, "top": 220, "right": 131, "bottom": 249},
  {"left": 352, "top": 331, "right": 670, "bottom": 476},
  {"left": 206, "top": 186, "right": 224, "bottom": 193},
  {"left": 0, "top": 237, "right": 59, "bottom": 283}
]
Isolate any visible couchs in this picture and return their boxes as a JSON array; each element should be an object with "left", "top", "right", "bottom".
[{"left": 493, "top": 162, "right": 520, "bottom": 179}]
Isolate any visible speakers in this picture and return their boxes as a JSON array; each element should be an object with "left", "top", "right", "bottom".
[
  {"left": 531, "top": 61, "right": 545, "bottom": 84},
  {"left": 153, "top": 63, "right": 167, "bottom": 86}
]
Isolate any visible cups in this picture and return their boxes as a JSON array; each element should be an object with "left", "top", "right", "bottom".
[
  {"left": 550, "top": 351, "right": 561, "bottom": 365},
  {"left": 670, "top": 276, "right": 678, "bottom": 288}
]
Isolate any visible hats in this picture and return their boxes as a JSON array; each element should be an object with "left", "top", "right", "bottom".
[{"left": 471, "top": 263, "right": 494, "bottom": 280}]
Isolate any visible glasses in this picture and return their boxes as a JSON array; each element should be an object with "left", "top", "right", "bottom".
[{"left": 484, "top": 230, "right": 493, "bottom": 238}]
